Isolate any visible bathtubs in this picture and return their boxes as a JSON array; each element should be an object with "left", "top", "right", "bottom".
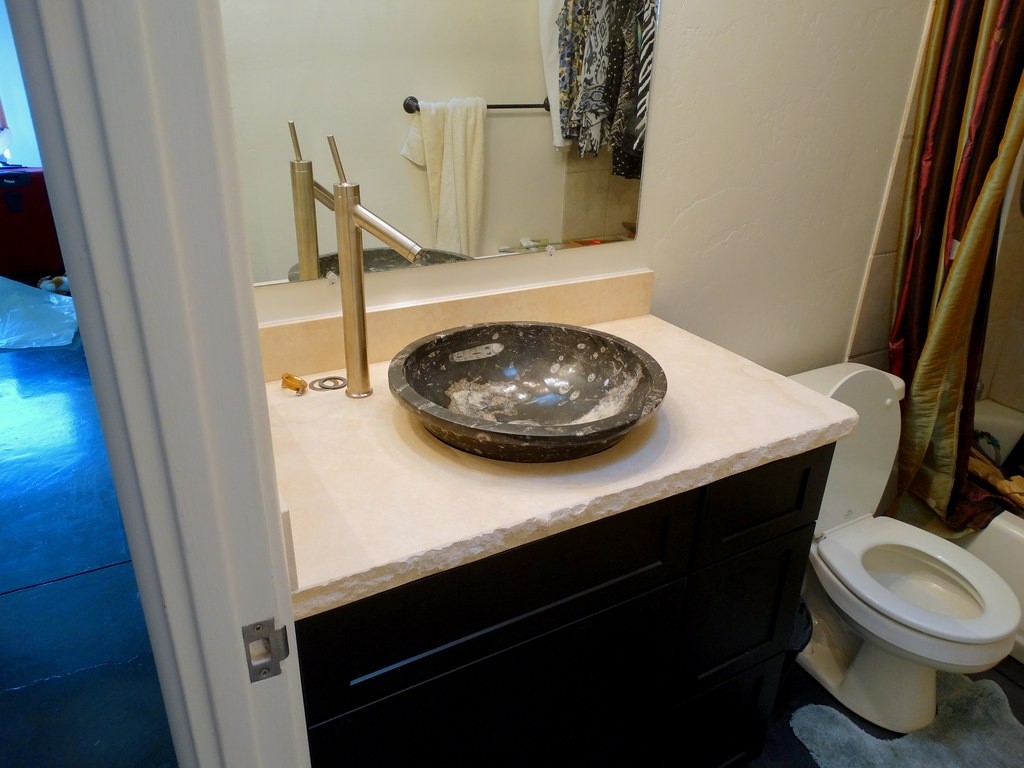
[{"left": 944, "top": 397, "right": 1022, "bottom": 666}]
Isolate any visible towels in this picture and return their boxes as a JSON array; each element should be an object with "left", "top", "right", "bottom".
[{"left": 398, "top": 97, "right": 487, "bottom": 257}]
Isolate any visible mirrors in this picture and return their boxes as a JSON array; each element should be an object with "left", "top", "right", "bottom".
[{"left": 219, "top": 0, "right": 660, "bottom": 288}]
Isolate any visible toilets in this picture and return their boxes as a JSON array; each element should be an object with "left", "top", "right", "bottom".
[{"left": 744, "top": 363, "right": 1022, "bottom": 733}]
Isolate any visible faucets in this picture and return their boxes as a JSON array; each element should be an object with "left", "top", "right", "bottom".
[
  {"left": 287, "top": 121, "right": 335, "bottom": 280},
  {"left": 327, "top": 134, "right": 423, "bottom": 398}
]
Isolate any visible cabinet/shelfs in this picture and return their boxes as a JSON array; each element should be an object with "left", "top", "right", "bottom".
[{"left": 294, "top": 440, "right": 837, "bottom": 768}]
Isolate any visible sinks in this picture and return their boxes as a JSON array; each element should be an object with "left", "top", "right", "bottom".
[
  {"left": 287, "top": 246, "right": 476, "bottom": 283},
  {"left": 388, "top": 322, "right": 667, "bottom": 463}
]
